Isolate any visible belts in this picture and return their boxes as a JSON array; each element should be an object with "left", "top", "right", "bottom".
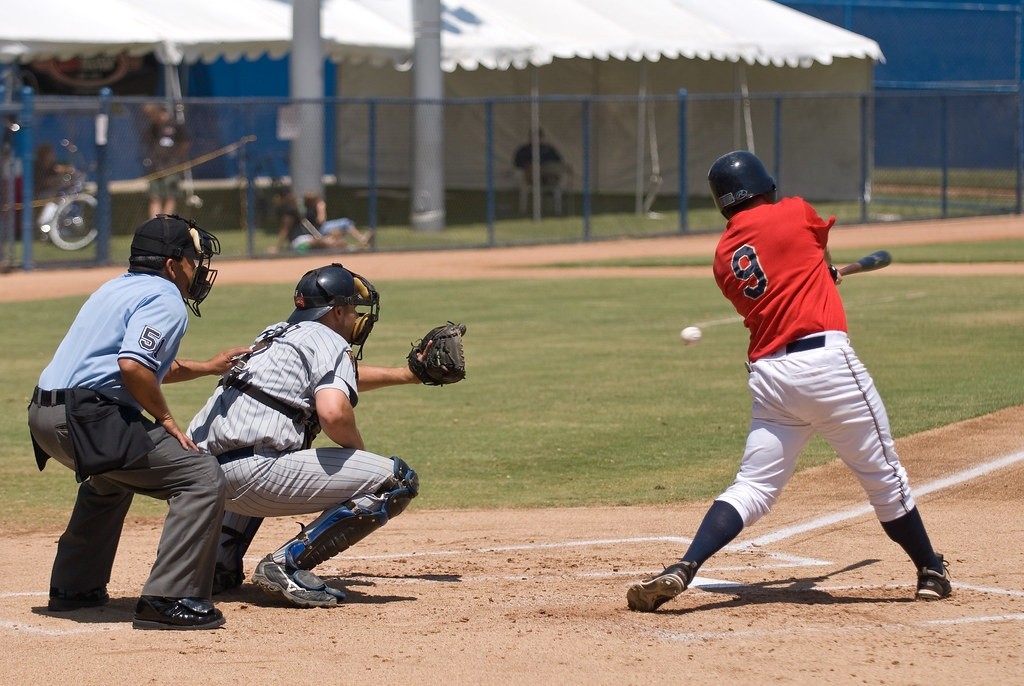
[
  {"left": 32, "top": 385, "right": 68, "bottom": 407},
  {"left": 784, "top": 336, "right": 826, "bottom": 354},
  {"left": 215, "top": 444, "right": 253, "bottom": 465}
]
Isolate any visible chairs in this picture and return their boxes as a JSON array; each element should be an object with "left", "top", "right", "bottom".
[{"left": 515, "top": 161, "right": 568, "bottom": 218}]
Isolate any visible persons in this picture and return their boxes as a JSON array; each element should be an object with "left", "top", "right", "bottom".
[
  {"left": 0, "top": 139, "right": 24, "bottom": 239},
  {"left": 270, "top": 192, "right": 373, "bottom": 251},
  {"left": 34, "top": 144, "right": 79, "bottom": 197},
  {"left": 512, "top": 125, "right": 574, "bottom": 217},
  {"left": 188, "top": 262, "right": 464, "bottom": 607},
  {"left": 627, "top": 149, "right": 953, "bottom": 611},
  {"left": 140, "top": 107, "right": 190, "bottom": 218},
  {"left": 27, "top": 214, "right": 253, "bottom": 629}
]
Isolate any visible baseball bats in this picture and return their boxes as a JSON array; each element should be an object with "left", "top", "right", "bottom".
[{"left": 838, "top": 249, "right": 892, "bottom": 277}]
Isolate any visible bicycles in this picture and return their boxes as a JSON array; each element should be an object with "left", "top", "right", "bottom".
[{"left": 36, "top": 137, "right": 103, "bottom": 253}]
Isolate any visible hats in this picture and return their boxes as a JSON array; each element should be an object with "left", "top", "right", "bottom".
[{"left": 129, "top": 217, "right": 212, "bottom": 258}]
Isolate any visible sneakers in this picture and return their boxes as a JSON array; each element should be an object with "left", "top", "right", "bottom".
[
  {"left": 208, "top": 572, "right": 242, "bottom": 594},
  {"left": 249, "top": 552, "right": 337, "bottom": 608},
  {"left": 626, "top": 559, "right": 699, "bottom": 613},
  {"left": 915, "top": 552, "right": 953, "bottom": 600}
]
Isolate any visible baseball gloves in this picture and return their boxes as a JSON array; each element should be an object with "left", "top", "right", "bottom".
[{"left": 408, "top": 322, "right": 467, "bottom": 386}]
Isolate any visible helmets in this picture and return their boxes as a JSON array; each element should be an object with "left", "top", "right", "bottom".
[
  {"left": 708, "top": 151, "right": 777, "bottom": 221},
  {"left": 286, "top": 266, "right": 355, "bottom": 323}
]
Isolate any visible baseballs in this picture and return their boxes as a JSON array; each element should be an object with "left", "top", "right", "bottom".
[{"left": 680, "top": 326, "right": 703, "bottom": 344}]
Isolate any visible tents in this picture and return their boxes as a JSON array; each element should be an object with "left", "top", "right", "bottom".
[{"left": 0, "top": 0, "right": 882, "bottom": 203}]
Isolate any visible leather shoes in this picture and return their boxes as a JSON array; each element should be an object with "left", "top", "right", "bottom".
[
  {"left": 47, "top": 584, "right": 110, "bottom": 612},
  {"left": 132, "top": 595, "right": 227, "bottom": 631}
]
